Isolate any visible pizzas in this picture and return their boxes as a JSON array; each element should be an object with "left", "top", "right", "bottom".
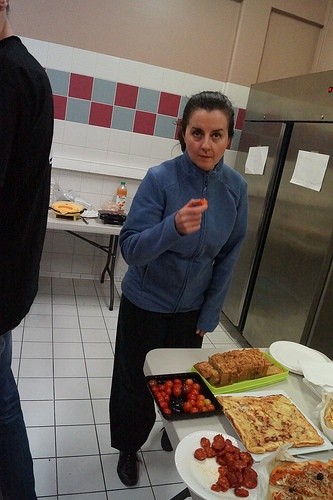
[{"left": 269, "top": 459, "right": 333, "bottom": 500}]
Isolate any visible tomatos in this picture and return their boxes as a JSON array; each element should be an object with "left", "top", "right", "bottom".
[
  {"left": 150, "top": 379, "right": 215, "bottom": 414},
  {"left": 199, "top": 198, "right": 208, "bottom": 205}
]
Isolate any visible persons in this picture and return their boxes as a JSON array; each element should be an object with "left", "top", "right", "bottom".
[
  {"left": 1, "top": 0, "right": 57, "bottom": 500},
  {"left": 108, "top": 92, "right": 250, "bottom": 488}
]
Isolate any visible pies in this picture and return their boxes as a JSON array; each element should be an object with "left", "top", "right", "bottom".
[
  {"left": 216, "top": 394, "right": 324, "bottom": 454},
  {"left": 324, "top": 405, "right": 333, "bottom": 429}
]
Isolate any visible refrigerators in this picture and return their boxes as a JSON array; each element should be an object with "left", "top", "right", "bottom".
[{"left": 219, "top": 69, "right": 333, "bottom": 362}]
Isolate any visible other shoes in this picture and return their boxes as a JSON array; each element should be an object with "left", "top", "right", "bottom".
[
  {"left": 159, "top": 426, "right": 174, "bottom": 451},
  {"left": 117, "top": 450, "right": 141, "bottom": 486}
]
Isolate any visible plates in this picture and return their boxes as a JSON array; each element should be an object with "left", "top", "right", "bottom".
[
  {"left": 268, "top": 340, "right": 333, "bottom": 375},
  {"left": 175, "top": 431, "right": 260, "bottom": 500},
  {"left": 145, "top": 372, "right": 224, "bottom": 421},
  {"left": 213, "top": 390, "right": 333, "bottom": 462}
]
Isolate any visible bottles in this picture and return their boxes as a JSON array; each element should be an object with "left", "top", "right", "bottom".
[{"left": 114, "top": 180, "right": 127, "bottom": 214}]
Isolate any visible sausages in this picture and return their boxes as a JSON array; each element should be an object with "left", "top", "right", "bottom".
[{"left": 194, "top": 434, "right": 258, "bottom": 497}]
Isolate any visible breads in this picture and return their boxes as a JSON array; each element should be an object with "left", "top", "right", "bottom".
[{"left": 194, "top": 348, "right": 283, "bottom": 386}]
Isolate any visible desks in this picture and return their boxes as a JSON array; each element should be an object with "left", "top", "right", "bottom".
[
  {"left": 46, "top": 209, "right": 123, "bottom": 311},
  {"left": 145, "top": 348, "right": 333, "bottom": 500}
]
